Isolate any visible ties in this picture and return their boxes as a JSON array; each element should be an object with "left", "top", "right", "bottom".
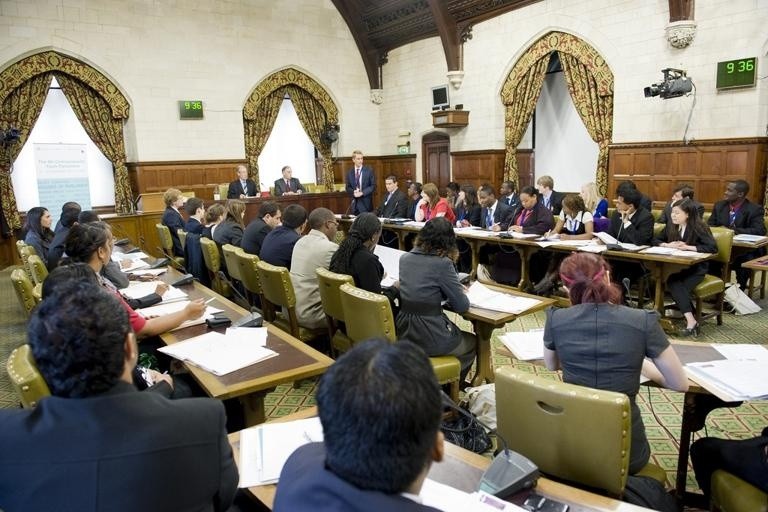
[
  {"left": 243, "top": 181, "right": 248, "bottom": 197},
  {"left": 355, "top": 168, "right": 360, "bottom": 189},
  {"left": 286, "top": 180, "right": 290, "bottom": 192},
  {"left": 729, "top": 206, "right": 737, "bottom": 228}
]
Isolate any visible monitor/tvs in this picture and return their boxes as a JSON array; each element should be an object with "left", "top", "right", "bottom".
[{"left": 431, "top": 84, "right": 449, "bottom": 113}]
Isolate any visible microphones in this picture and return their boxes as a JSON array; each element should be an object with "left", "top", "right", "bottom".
[
  {"left": 499, "top": 207, "right": 521, "bottom": 239},
  {"left": 384, "top": 201, "right": 399, "bottom": 224},
  {"left": 218, "top": 270, "right": 262, "bottom": 328},
  {"left": 623, "top": 278, "right": 634, "bottom": 308},
  {"left": 139, "top": 236, "right": 167, "bottom": 268},
  {"left": 439, "top": 390, "right": 539, "bottom": 497},
  {"left": 341, "top": 200, "right": 355, "bottom": 219},
  {"left": 156, "top": 247, "right": 193, "bottom": 287},
  {"left": 606, "top": 213, "right": 627, "bottom": 251}
]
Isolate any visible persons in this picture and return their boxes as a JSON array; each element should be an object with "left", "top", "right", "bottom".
[
  {"left": 455, "top": 185, "right": 481, "bottom": 228},
  {"left": 610, "top": 189, "right": 654, "bottom": 292},
  {"left": 40, "top": 263, "right": 189, "bottom": 398},
  {"left": 690, "top": 427, "right": 768, "bottom": 501},
  {"left": 543, "top": 251, "right": 689, "bottom": 477},
  {"left": 534, "top": 194, "right": 594, "bottom": 295},
  {"left": 616, "top": 181, "right": 652, "bottom": 210},
  {"left": 445, "top": 181, "right": 460, "bottom": 205},
  {"left": 274, "top": 166, "right": 306, "bottom": 196},
  {"left": 328, "top": 212, "right": 399, "bottom": 339},
  {"left": 282, "top": 207, "right": 340, "bottom": 331},
  {"left": 241, "top": 201, "right": 281, "bottom": 259},
  {"left": 414, "top": 183, "right": 455, "bottom": 226},
  {"left": 0, "top": 278, "right": 240, "bottom": 512},
  {"left": 536, "top": 175, "right": 565, "bottom": 215},
  {"left": 213, "top": 199, "right": 245, "bottom": 273},
  {"left": 78, "top": 210, "right": 158, "bottom": 289},
  {"left": 201, "top": 203, "right": 224, "bottom": 241},
  {"left": 227, "top": 165, "right": 261, "bottom": 199},
  {"left": 377, "top": 175, "right": 407, "bottom": 219},
  {"left": 407, "top": 182, "right": 422, "bottom": 221},
  {"left": 183, "top": 197, "right": 205, "bottom": 235},
  {"left": 64, "top": 222, "right": 207, "bottom": 337},
  {"left": 479, "top": 186, "right": 514, "bottom": 232},
  {"left": 272, "top": 336, "right": 446, "bottom": 512},
  {"left": 48, "top": 209, "right": 81, "bottom": 269},
  {"left": 396, "top": 217, "right": 479, "bottom": 388},
  {"left": 259, "top": 204, "right": 308, "bottom": 272},
  {"left": 652, "top": 199, "right": 718, "bottom": 337},
  {"left": 657, "top": 185, "right": 705, "bottom": 224},
  {"left": 23, "top": 207, "right": 54, "bottom": 267},
  {"left": 498, "top": 180, "right": 520, "bottom": 209},
  {"left": 707, "top": 179, "right": 767, "bottom": 292},
  {"left": 54, "top": 201, "right": 81, "bottom": 237},
  {"left": 162, "top": 188, "right": 186, "bottom": 256},
  {"left": 508, "top": 186, "right": 555, "bottom": 235},
  {"left": 346, "top": 151, "right": 376, "bottom": 215}
]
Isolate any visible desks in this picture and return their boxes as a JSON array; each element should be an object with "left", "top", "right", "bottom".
[
  {"left": 445, "top": 260, "right": 559, "bottom": 387},
  {"left": 329, "top": 211, "right": 718, "bottom": 332},
  {"left": 99, "top": 240, "right": 334, "bottom": 426},
  {"left": 97, "top": 190, "right": 352, "bottom": 255},
  {"left": 228, "top": 403, "right": 651, "bottom": 512},
  {"left": 740, "top": 116, "right": 768, "bottom": 255},
  {"left": 494, "top": 322, "right": 768, "bottom": 511}
]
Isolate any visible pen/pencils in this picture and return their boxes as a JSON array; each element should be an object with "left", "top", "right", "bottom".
[
  {"left": 204, "top": 296, "right": 217, "bottom": 305},
  {"left": 546, "top": 228, "right": 552, "bottom": 239},
  {"left": 138, "top": 275, "right": 159, "bottom": 279},
  {"left": 493, "top": 221, "right": 500, "bottom": 227}
]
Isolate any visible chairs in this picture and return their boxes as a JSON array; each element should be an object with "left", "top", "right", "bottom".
[
  {"left": 32, "top": 281, "right": 44, "bottom": 302},
  {"left": 306, "top": 185, "right": 327, "bottom": 193},
  {"left": 20, "top": 247, "right": 35, "bottom": 273},
  {"left": 15, "top": 239, "right": 26, "bottom": 254},
  {"left": 650, "top": 221, "right": 668, "bottom": 236},
  {"left": 605, "top": 207, "right": 617, "bottom": 216},
  {"left": 703, "top": 210, "right": 713, "bottom": 222},
  {"left": 182, "top": 191, "right": 196, "bottom": 198},
  {"left": 688, "top": 227, "right": 737, "bottom": 334},
  {"left": 492, "top": 364, "right": 666, "bottom": 504},
  {"left": 11, "top": 269, "right": 33, "bottom": 317},
  {"left": 176, "top": 227, "right": 190, "bottom": 252},
  {"left": 217, "top": 183, "right": 230, "bottom": 200},
  {"left": 233, "top": 248, "right": 260, "bottom": 318},
  {"left": 269, "top": 186, "right": 276, "bottom": 195},
  {"left": 6, "top": 343, "right": 52, "bottom": 409},
  {"left": 154, "top": 222, "right": 185, "bottom": 270},
  {"left": 314, "top": 265, "right": 354, "bottom": 357},
  {"left": 332, "top": 184, "right": 346, "bottom": 192},
  {"left": 256, "top": 260, "right": 329, "bottom": 389},
  {"left": 338, "top": 282, "right": 462, "bottom": 418},
  {"left": 198, "top": 236, "right": 229, "bottom": 296},
  {"left": 27, "top": 254, "right": 49, "bottom": 285},
  {"left": 650, "top": 208, "right": 664, "bottom": 219},
  {"left": 221, "top": 242, "right": 244, "bottom": 306},
  {"left": 551, "top": 214, "right": 561, "bottom": 224}
]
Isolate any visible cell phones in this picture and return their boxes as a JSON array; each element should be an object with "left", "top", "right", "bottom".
[{"left": 523, "top": 495, "right": 569, "bottom": 512}]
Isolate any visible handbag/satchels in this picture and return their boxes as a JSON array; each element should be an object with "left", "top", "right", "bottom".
[
  {"left": 459, "top": 382, "right": 497, "bottom": 434},
  {"left": 723, "top": 283, "right": 763, "bottom": 316},
  {"left": 439, "top": 408, "right": 493, "bottom": 454}
]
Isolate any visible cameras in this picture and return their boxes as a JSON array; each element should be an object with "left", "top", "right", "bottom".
[{"left": 644, "top": 68, "right": 692, "bottom": 100}]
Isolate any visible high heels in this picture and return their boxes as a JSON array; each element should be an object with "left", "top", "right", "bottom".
[{"left": 682, "top": 321, "right": 700, "bottom": 337}]
